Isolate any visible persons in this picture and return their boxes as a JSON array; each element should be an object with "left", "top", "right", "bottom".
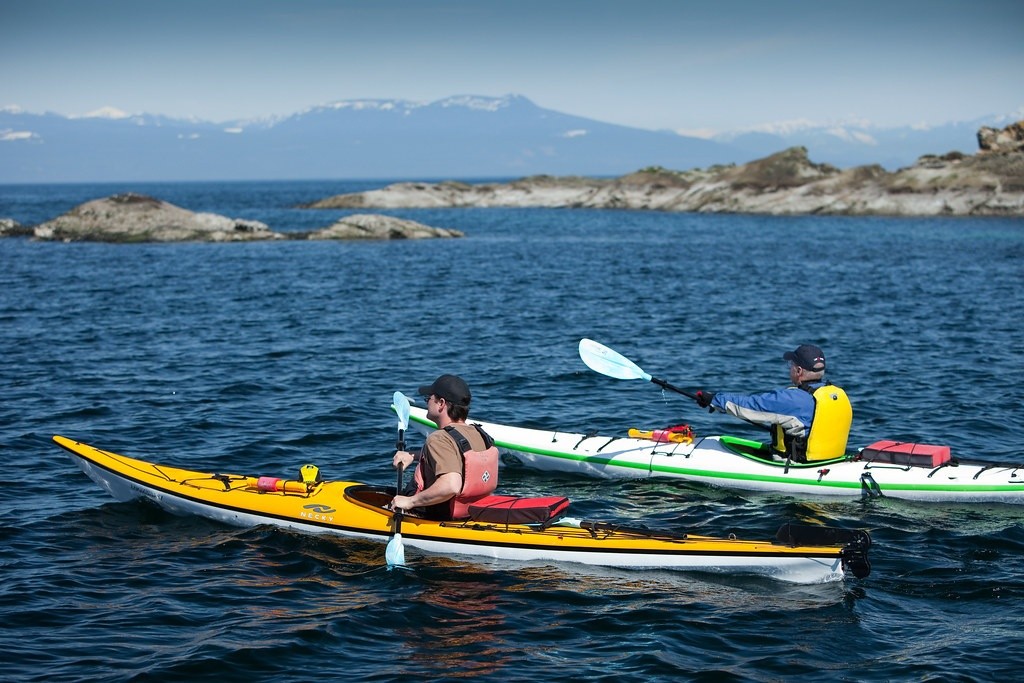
[
  {"left": 381, "top": 374, "right": 499, "bottom": 522},
  {"left": 696, "top": 345, "right": 853, "bottom": 464}
]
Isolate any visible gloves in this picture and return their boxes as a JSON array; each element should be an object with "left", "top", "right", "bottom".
[{"left": 696, "top": 390, "right": 717, "bottom": 413}]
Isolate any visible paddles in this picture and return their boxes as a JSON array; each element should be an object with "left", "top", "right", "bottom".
[
  {"left": 387, "top": 391, "right": 409, "bottom": 566},
  {"left": 577, "top": 338, "right": 864, "bottom": 456}
]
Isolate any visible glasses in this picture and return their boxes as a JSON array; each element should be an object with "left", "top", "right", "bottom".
[{"left": 424, "top": 396, "right": 435, "bottom": 402}]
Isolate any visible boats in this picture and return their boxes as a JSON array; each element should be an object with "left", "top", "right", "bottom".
[
  {"left": 51, "top": 433, "right": 846, "bottom": 589},
  {"left": 390, "top": 401, "right": 1023, "bottom": 502}
]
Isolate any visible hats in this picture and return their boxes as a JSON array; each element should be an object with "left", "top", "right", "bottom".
[
  {"left": 419, "top": 374, "right": 472, "bottom": 406},
  {"left": 784, "top": 344, "right": 825, "bottom": 372}
]
